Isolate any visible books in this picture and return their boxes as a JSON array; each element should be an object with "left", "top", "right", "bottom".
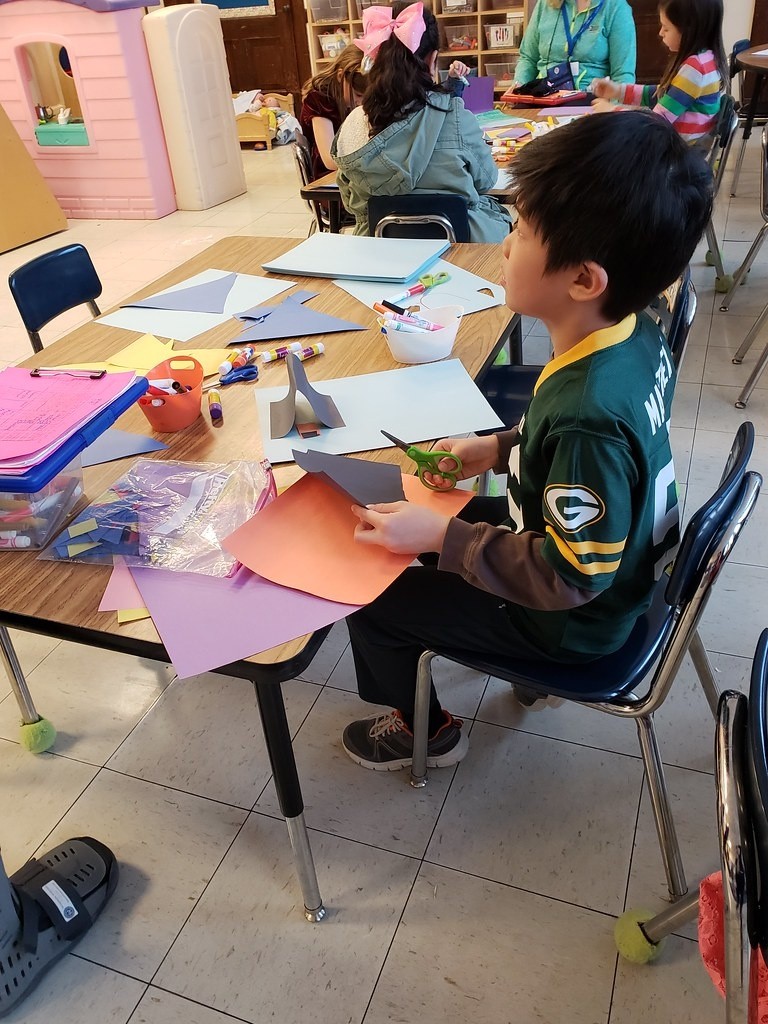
[
  {"left": 0, "top": 365, "right": 138, "bottom": 476},
  {"left": 260, "top": 232, "right": 452, "bottom": 283}
]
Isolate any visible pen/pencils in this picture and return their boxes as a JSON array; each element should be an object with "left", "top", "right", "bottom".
[{"left": 34, "top": 477, "right": 79, "bottom": 548}]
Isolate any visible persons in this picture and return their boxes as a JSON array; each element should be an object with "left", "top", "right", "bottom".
[
  {"left": 340, "top": 109, "right": 716, "bottom": 771},
  {"left": 591, "top": 0, "right": 730, "bottom": 159},
  {"left": 511, "top": 0, "right": 637, "bottom": 109},
  {"left": 329, "top": 0, "right": 514, "bottom": 243},
  {"left": 231, "top": 92, "right": 280, "bottom": 130},
  {"left": 299, "top": 42, "right": 371, "bottom": 220},
  {"left": 442, "top": 60, "right": 471, "bottom": 98}
]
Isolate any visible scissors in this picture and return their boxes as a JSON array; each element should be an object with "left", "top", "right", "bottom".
[
  {"left": 202, "top": 364, "right": 259, "bottom": 391},
  {"left": 420, "top": 272, "right": 449, "bottom": 295},
  {"left": 381, "top": 430, "right": 462, "bottom": 492}
]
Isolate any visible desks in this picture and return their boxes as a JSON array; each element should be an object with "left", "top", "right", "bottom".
[
  {"left": 730, "top": 44, "right": 768, "bottom": 198},
  {"left": 1, "top": 237, "right": 534, "bottom": 922},
  {"left": 301, "top": 104, "right": 648, "bottom": 242}
]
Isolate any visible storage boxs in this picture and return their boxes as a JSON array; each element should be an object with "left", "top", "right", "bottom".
[
  {"left": 439, "top": 66, "right": 478, "bottom": 85},
  {"left": 1, "top": 451, "right": 84, "bottom": 553},
  {"left": 354, "top": 0, "right": 392, "bottom": 23},
  {"left": 318, "top": 32, "right": 350, "bottom": 59},
  {"left": 442, "top": 0, "right": 476, "bottom": 14},
  {"left": 444, "top": 24, "right": 477, "bottom": 50},
  {"left": 484, "top": 62, "right": 518, "bottom": 86},
  {"left": 483, "top": 23, "right": 522, "bottom": 50},
  {"left": 305, "top": 0, "right": 349, "bottom": 24}
]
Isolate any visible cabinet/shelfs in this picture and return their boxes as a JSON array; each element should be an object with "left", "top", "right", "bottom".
[{"left": 304, "top": 0, "right": 530, "bottom": 93}]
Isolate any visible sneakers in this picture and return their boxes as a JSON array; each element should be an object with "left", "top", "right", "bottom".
[
  {"left": 510, "top": 682, "right": 565, "bottom": 712},
  {"left": 342, "top": 709, "right": 470, "bottom": 770}
]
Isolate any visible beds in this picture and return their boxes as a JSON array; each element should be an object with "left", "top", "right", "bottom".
[{"left": 228, "top": 89, "right": 293, "bottom": 150}]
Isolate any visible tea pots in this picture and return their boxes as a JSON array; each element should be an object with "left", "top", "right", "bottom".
[{"left": 35, "top": 103, "right": 53, "bottom": 123}]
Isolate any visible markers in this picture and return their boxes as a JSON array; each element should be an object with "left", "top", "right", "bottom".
[
  {"left": 147, "top": 386, "right": 168, "bottom": 396},
  {"left": 525, "top": 115, "right": 554, "bottom": 137},
  {"left": 0, "top": 475, "right": 81, "bottom": 548},
  {"left": 584, "top": 76, "right": 610, "bottom": 92},
  {"left": 173, "top": 381, "right": 189, "bottom": 393},
  {"left": 373, "top": 284, "right": 444, "bottom": 334}
]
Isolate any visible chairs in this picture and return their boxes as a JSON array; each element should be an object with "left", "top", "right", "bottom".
[{"left": 24, "top": 40, "right": 768, "bottom": 1024}]
